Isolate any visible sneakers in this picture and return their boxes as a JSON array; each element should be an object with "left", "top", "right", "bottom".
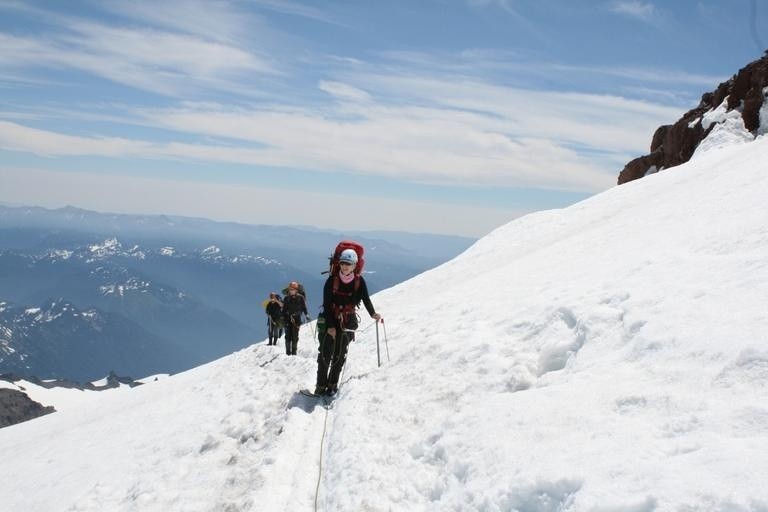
[{"left": 312, "top": 380, "right": 340, "bottom": 397}]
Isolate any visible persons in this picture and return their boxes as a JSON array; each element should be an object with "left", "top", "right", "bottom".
[
  {"left": 312, "top": 249, "right": 384, "bottom": 398},
  {"left": 264, "top": 292, "right": 283, "bottom": 345},
  {"left": 281, "top": 282, "right": 311, "bottom": 355}
]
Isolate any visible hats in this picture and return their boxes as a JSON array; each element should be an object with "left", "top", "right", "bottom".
[
  {"left": 338, "top": 247, "right": 360, "bottom": 264},
  {"left": 286, "top": 280, "right": 299, "bottom": 289}
]
